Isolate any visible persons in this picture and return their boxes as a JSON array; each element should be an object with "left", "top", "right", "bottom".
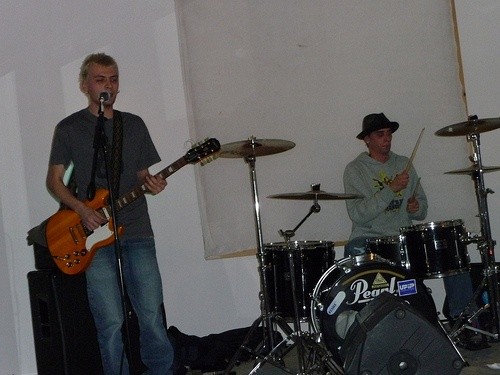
[
  {"left": 44, "top": 52, "right": 176, "bottom": 375},
  {"left": 342, "top": 112, "right": 487, "bottom": 350}
]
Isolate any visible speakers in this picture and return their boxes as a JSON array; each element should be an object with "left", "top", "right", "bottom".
[
  {"left": 338, "top": 291, "right": 463, "bottom": 375},
  {"left": 28, "top": 270, "right": 167, "bottom": 375}
]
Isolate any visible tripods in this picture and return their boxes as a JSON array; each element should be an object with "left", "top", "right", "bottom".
[
  {"left": 224, "top": 145, "right": 344, "bottom": 375},
  {"left": 448, "top": 172, "right": 500, "bottom": 342}
]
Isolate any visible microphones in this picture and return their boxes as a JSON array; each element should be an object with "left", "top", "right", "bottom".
[
  {"left": 99, "top": 92, "right": 110, "bottom": 102},
  {"left": 459, "top": 237, "right": 485, "bottom": 244}
]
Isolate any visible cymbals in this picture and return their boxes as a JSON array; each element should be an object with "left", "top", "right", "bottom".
[
  {"left": 218, "top": 138, "right": 296, "bottom": 160},
  {"left": 264, "top": 190, "right": 366, "bottom": 201},
  {"left": 442, "top": 165, "right": 500, "bottom": 176},
  {"left": 434, "top": 117, "right": 500, "bottom": 138}
]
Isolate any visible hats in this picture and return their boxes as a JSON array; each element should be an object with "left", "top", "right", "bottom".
[{"left": 355, "top": 113, "right": 399, "bottom": 140}]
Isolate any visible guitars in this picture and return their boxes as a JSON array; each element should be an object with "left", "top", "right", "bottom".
[{"left": 45, "top": 135, "right": 222, "bottom": 276}]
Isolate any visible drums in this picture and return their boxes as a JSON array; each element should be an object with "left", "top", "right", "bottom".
[
  {"left": 261, "top": 239, "right": 336, "bottom": 324},
  {"left": 399, "top": 218, "right": 473, "bottom": 280},
  {"left": 308, "top": 254, "right": 439, "bottom": 366},
  {"left": 345, "top": 234, "right": 411, "bottom": 269}
]
